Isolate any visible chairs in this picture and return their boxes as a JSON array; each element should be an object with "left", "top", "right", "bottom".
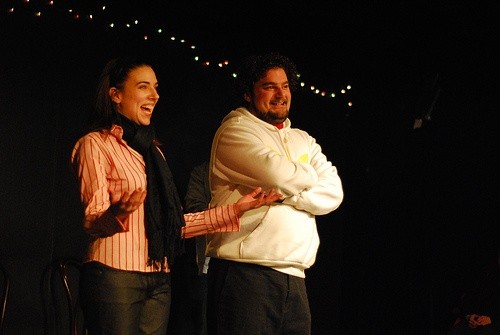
[
  {"left": 59, "top": 257, "right": 90, "bottom": 335},
  {"left": 0, "top": 267, "right": 10, "bottom": 335}
]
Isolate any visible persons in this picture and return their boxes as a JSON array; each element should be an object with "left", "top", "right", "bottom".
[
  {"left": 206, "top": 51, "right": 345, "bottom": 335},
  {"left": 68, "top": 53, "right": 282, "bottom": 335}
]
